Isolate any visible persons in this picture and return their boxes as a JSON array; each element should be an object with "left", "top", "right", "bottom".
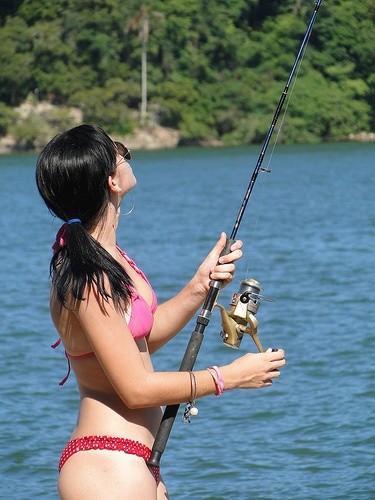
[{"left": 37, "top": 124, "right": 286, "bottom": 500}]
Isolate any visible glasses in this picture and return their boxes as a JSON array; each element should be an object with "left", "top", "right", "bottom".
[{"left": 111, "top": 141, "right": 130, "bottom": 174}]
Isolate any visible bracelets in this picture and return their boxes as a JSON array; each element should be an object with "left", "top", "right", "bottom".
[
  {"left": 182, "top": 373, "right": 199, "bottom": 424},
  {"left": 207, "top": 365, "right": 223, "bottom": 396}
]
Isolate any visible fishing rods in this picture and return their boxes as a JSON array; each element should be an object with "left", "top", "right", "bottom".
[{"left": 146, "top": 0, "right": 322, "bottom": 468}]
